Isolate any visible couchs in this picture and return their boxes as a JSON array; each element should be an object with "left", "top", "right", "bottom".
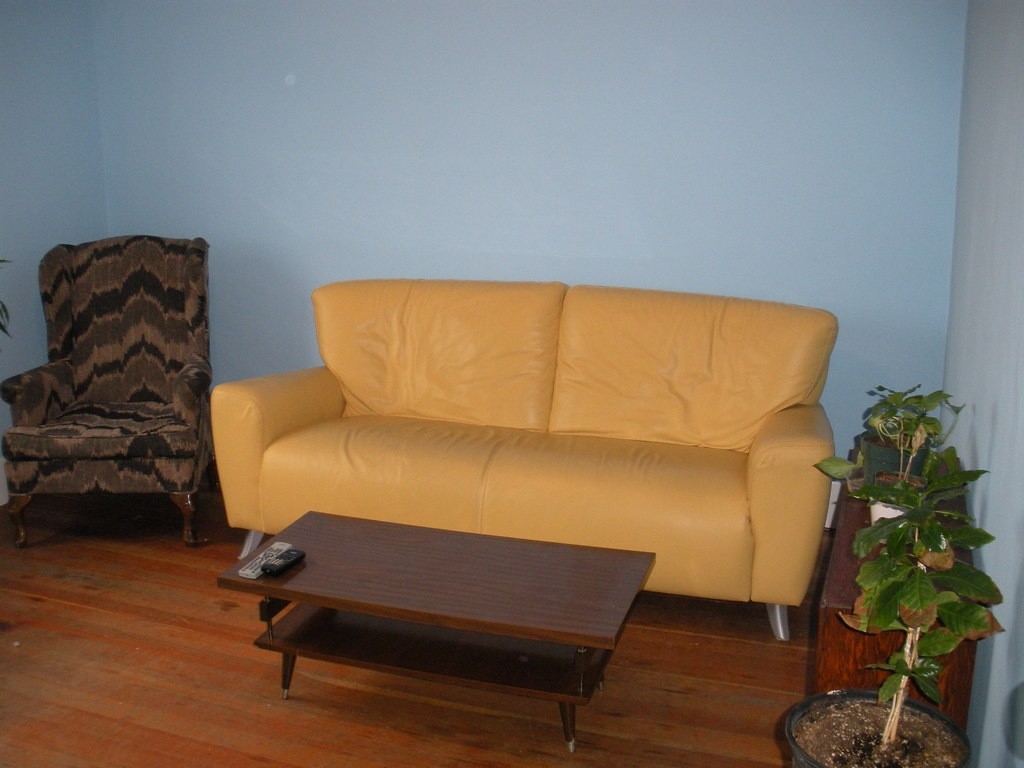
[{"left": 210, "top": 278, "right": 840, "bottom": 640}]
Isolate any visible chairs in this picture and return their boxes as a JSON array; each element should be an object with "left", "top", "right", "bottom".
[{"left": 0, "top": 234, "right": 212, "bottom": 548}]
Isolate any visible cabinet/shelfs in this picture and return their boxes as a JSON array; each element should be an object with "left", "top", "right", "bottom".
[{"left": 810, "top": 447, "right": 978, "bottom": 729}]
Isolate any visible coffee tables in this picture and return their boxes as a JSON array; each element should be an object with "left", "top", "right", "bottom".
[{"left": 216, "top": 510, "right": 657, "bottom": 752}]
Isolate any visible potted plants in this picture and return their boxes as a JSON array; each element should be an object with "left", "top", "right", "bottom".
[
  {"left": 868, "top": 415, "right": 928, "bottom": 544},
  {"left": 813, "top": 384, "right": 953, "bottom": 498},
  {"left": 784, "top": 446, "right": 1006, "bottom": 768}
]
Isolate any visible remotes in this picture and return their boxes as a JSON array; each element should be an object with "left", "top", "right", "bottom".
[
  {"left": 238, "top": 542, "right": 292, "bottom": 579},
  {"left": 261, "top": 549, "right": 305, "bottom": 577}
]
